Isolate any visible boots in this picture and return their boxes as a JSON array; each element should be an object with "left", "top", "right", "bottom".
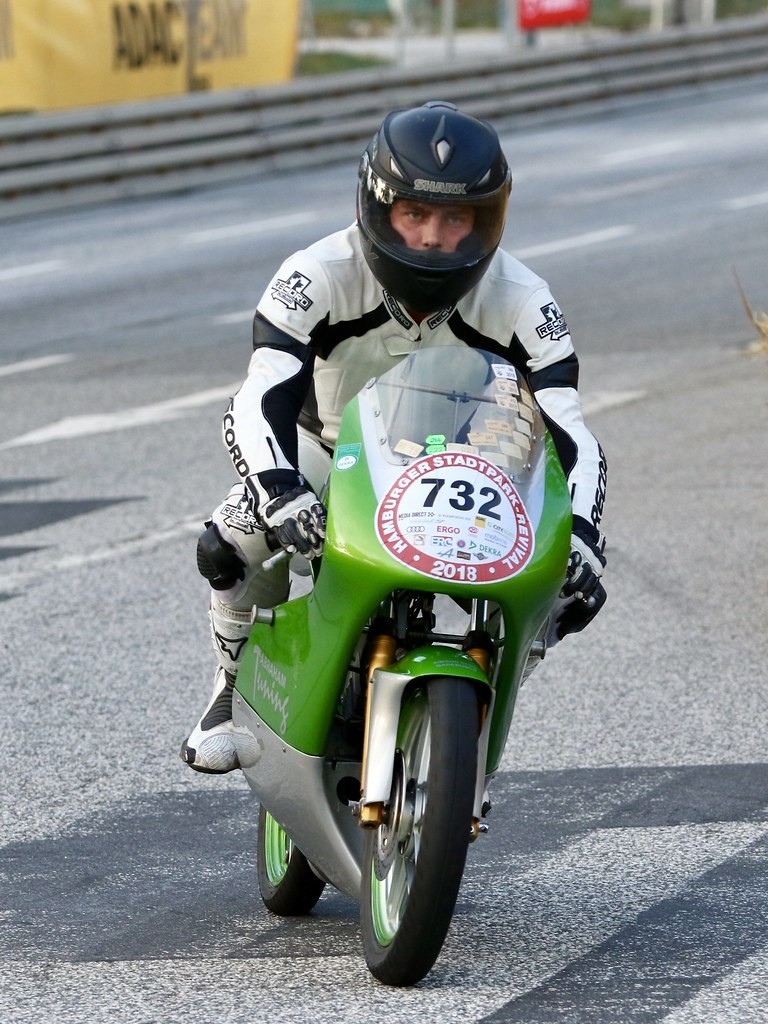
[{"left": 181, "top": 591, "right": 287, "bottom": 774}]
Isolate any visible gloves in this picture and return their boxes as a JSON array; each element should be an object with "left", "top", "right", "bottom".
[
  {"left": 244, "top": 469, "right": 327, "bottom": 560},
  {"left": 559, "top": 514, "right": 606, "bottom": 608}
]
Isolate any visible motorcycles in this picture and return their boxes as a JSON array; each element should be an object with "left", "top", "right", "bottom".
[{"left": 232, "top": 344, "right": 595, "bottom": 990}]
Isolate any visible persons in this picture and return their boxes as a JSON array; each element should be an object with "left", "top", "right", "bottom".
[{"left": 178, "top": 104, "right": 608, "bottom": 775}]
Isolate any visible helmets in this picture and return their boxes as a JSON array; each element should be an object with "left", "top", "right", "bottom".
[{"left": 357, "top": 101, "right": 513, "bottom": 311}]
